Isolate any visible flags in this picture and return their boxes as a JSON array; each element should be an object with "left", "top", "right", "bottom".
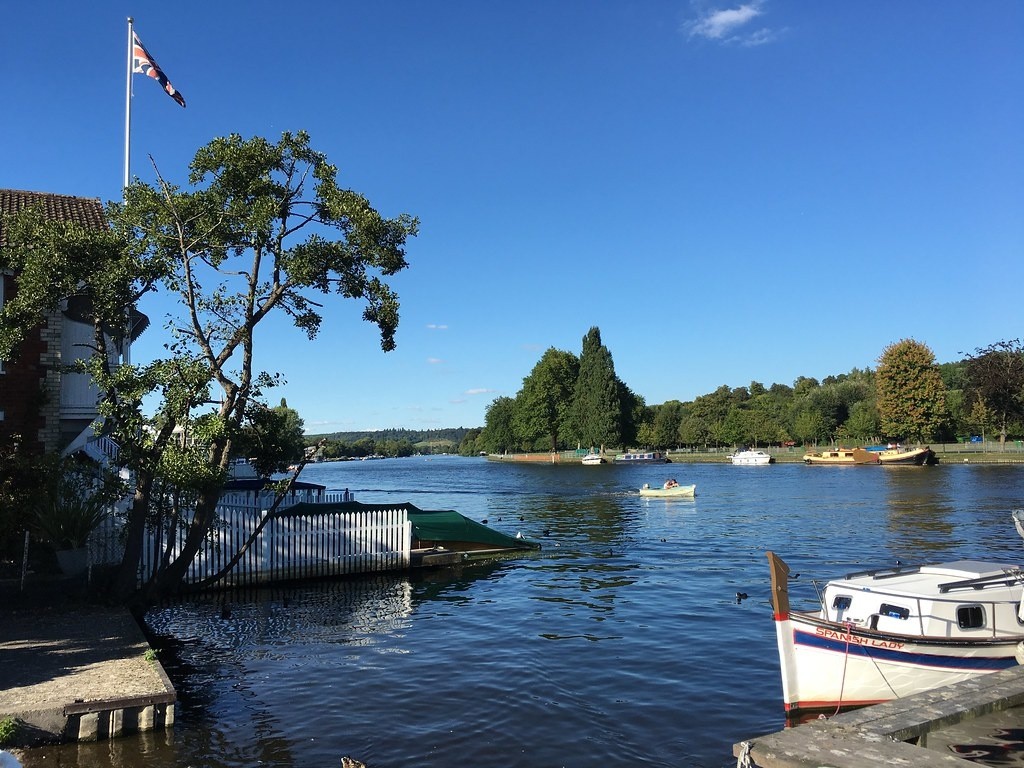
[{"left": 132, "top": 30, "right": 186, "bottom": 108}]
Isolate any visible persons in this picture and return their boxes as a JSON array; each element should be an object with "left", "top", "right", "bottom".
[{"left": 664, "top": 479, "right": 679, "bottom": 489}]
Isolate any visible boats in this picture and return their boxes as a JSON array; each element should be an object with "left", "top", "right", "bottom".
[
  {"left": 612, "top": 451, "right": 669, "bottom": 466},
  {"left": 802, "top": 443, "right": 937, "bottom": 464},
  {"left": 582, "top": 454, "right": 605, "bottom": 465},
  {"left": 768, "top": 550, "right": 1024, "bottom": 721},
  {"left": 638, "top": 484, "right": 696, "bottom": 498},
  {"left": 725, "top": 450, "right": 771, "bottom": 466}
]
